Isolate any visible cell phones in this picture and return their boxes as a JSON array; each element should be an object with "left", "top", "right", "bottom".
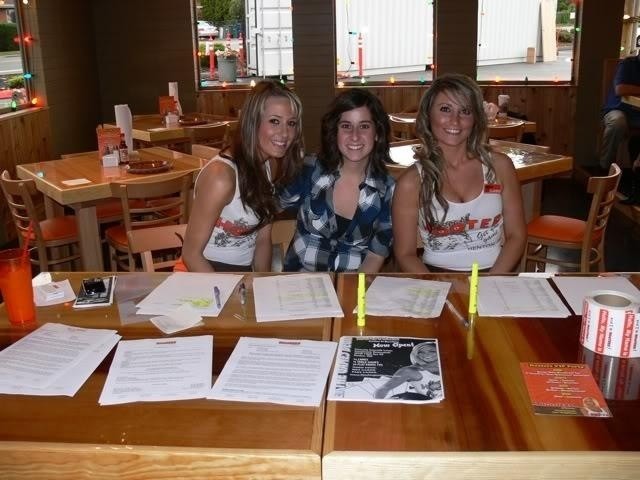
[{"left": 81, "top": 277, "right": 106, "bottom": 296}]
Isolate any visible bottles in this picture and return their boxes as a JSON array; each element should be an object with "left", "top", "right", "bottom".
[
  {"left": 160, "top": 110, "right": 173, "bottom": 125},
  {"left": 100, "top": 144, "right": 120, "bottom": 164},
  {"left": 172, "top": 100, "right": 179, "bottom": 114},
  {"left": 118, "top": 132, "right": 130, "bottom": 164}
]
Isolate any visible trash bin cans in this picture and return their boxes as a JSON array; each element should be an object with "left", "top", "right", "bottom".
[{"left": 218, "top": 57, "right": 236, "bottom": 81}]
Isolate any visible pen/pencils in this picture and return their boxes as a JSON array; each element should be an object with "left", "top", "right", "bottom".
[
  {"left": 214, "top": 286, "right": 221, "bottom": 309},
  {"left": 469, "top": 263, "right": 478, "bottom": 314},
  {"left": 239, "top": 283, "right": 246, "bottom": 306},
  {"left": 356, "top": 272, "right": 366, "bottom": 326},
  {"left": 445, "top": 298, "right": 470, "bottom": 327}
]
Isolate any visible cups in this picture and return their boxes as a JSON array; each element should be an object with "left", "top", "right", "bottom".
[
  {"left": 497, "top": 94, "right": 509, "bottom": 124},
  {"left": 1, "top": 248, "right": 33, "bottom": 326}
]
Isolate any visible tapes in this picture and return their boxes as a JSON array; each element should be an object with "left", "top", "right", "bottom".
[{"left": 580, "top": 289, "right": 640, "bottom": 360}]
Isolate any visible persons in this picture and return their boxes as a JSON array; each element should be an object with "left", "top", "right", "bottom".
[
  {"left": 599, "top": 49, "right": 640, "bottom": 176},
  {"left": 274, "top": 88, "right": 399, "bottom": 274},
  {"left": 581, "top": 397, "right": 610, "bottom": 417},
  {"left": 173, "top": 80, "right": 304, "bottom": 272},
  {"left": 392, "top": 72, "right": 528, "bottom": 273},
  {"left": 373, "top": 342, "right": 442, "bottom": 400}
]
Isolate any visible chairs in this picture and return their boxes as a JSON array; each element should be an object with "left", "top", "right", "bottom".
[
  {"left": 597, "top": 58, "right": 623, "bottom": 159},
  {"left": 486, "top": 123, "right": 526, "bottom": 143},
  {"left": 521, "top": 162, "right": 623, "bottom": 273},
  {"left": 251, "top": 220, "right": 298, "bottom": 272},
  {"left": 0, "top": 108, "right": 244, "bottom": 273},
  {"left": 388, "top": 119, "right": 417, "bottom": 142}
]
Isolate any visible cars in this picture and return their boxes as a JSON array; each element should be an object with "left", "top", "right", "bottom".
[
  {"left": 197, "top": 20, "right": 219, "bottom": 39},
  {"left": 636, "top": 26, "right": 640, "bottom": 48},
  {"left": 0, "top": 78, "right": 26, "bottom": 99}
]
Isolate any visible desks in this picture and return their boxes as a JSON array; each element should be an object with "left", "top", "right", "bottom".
[
  {"left": 383, "top": 136, "right": 574, "bottom": 225},
  {"left": 320, "top": 272, "right": 640, "bottom": 480},
  {"left": 388, "top": 110, "right": 536, "bottom": 143},
  {"left": 620, "top": 95, "right": 640, "bottom": 108},
  {"left": 0, "top": 269, "right": 334, "bottom": 479}
]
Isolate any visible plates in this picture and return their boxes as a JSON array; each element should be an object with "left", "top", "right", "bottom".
[
  {"left": 178, "top": 117, "right": 208, "bottom": 126},
  {"left": 125, "top": 159, "right": 176, "bottom": 175}
]
